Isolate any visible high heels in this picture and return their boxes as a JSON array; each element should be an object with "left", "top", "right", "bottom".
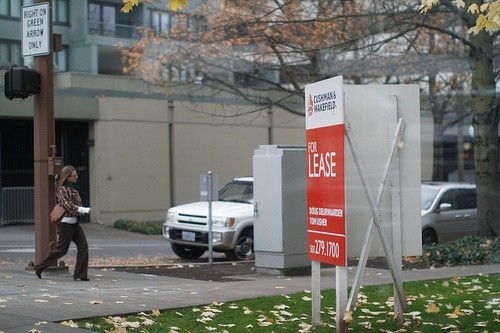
[
  {"left": 74, "top": 278, "right": 90, "bottom": 281},
  {"left": 34, "top": 266, "right": 42, "bottom": 279}
]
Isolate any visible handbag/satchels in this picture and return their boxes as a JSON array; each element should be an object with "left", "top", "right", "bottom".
[{"left": 50, "top": 204, "right": 66, "bottom": 223}]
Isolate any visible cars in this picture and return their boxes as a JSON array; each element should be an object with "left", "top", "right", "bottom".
[{"left": 421, "top": 180, "right": 478, "bottom": 246}]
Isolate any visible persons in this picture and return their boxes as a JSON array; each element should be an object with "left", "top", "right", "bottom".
[{"left": 34, "top": 166, "right": 93, "bottom": 281}]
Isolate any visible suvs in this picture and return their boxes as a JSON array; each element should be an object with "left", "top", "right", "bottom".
[{"left": 161, "top": 176, "right": 255, "bottom": 261}]
